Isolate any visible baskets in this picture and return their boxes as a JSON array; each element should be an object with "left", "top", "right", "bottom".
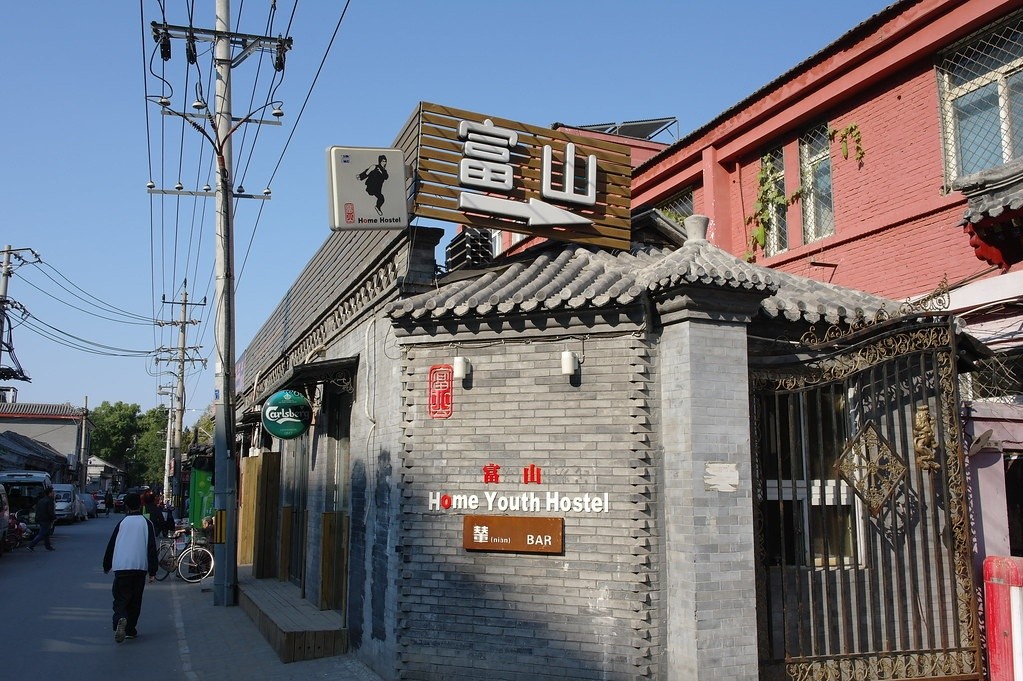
[{"left": 193, "top": 532, "right": 208, "bottom": 546}]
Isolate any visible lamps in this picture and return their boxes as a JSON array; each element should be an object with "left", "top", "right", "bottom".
[
  {"left": 561, "top": 351, "right": 579, "bottom": 375},
  {"left": 453, "top": 357, "right": 471, "bottom": 380}
]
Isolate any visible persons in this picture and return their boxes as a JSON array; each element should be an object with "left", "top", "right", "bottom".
[
  {"left": 141, "top": 489, "right": 153, "bottom": 514},
  {"left": 175, "top": 516, "right": 214, "bottom": 583},
  {"left": 104, "top": 489, "right": 113, "bottom": 518},
  {"left": 26, "top": 487, "right": 57, "bottom": 552},
  {"left": 102, "top": 493, "right": 158, "bottom": 643}
]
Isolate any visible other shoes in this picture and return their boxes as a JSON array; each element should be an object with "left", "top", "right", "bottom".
[
  {"left": 126, "top": 628, "right": 138, "bottom": 639},
  {"left": 44, "top": 547, "right": 56, "bottom": 552},
  {"left": 114, "top": 617, "right": 126, "bottom": 644},
  {"left": 26, "top": 547, "right": 35, "bottom": 552}
]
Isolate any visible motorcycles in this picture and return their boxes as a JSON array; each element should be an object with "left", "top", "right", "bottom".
[{"left": 10, "top": 510, "right": 43, "bottom": 542}]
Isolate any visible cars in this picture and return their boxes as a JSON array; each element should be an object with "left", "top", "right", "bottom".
[
  {"left": 76, "top": 494, "right": 99, "bottom": 522},
  {"left": 93, "top": 492, "right": 127, "bottom": 514}
]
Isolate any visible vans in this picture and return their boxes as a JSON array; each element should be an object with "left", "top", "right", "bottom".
[
  {"left": 51, "top": 484, "right": 77, "bottom": 524},
  {"left": 0, "top": 469, "right": 55, "bottom": 537},
  {"left": 0, "top": 484, "right": 10, "bottom": 554}
]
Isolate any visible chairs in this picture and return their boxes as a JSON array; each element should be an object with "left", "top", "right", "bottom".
[
  {"left": 10, "top": 489, "right": 21, "bottom": 496},
  {"left": 63, "top": 493, "right": 69, "bottom": 499},
  {"left": 56, "top": 494, "right": 61, "bottom": 499}
]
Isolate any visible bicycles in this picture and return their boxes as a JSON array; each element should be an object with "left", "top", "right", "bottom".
[
  {"left": 5, "top": 529, "right": 18, "bottom": 553},
  {"left": 154, "top": 523, "right": 214, "bottom": 583}
]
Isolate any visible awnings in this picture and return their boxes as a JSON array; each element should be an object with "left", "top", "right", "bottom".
[
  {"left": 249, "top": 356, "right": 358, "bottom": 405},
  {"left": 236, "top": 411, "right": 261, "bottom": 423},
  {"left": 236, "top": 424, "right": 252, "bottom": 433}
]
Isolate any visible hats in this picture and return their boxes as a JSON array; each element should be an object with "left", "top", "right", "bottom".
[{"left": 124, "top": 493, "right": 141, "bottom": 510}]
